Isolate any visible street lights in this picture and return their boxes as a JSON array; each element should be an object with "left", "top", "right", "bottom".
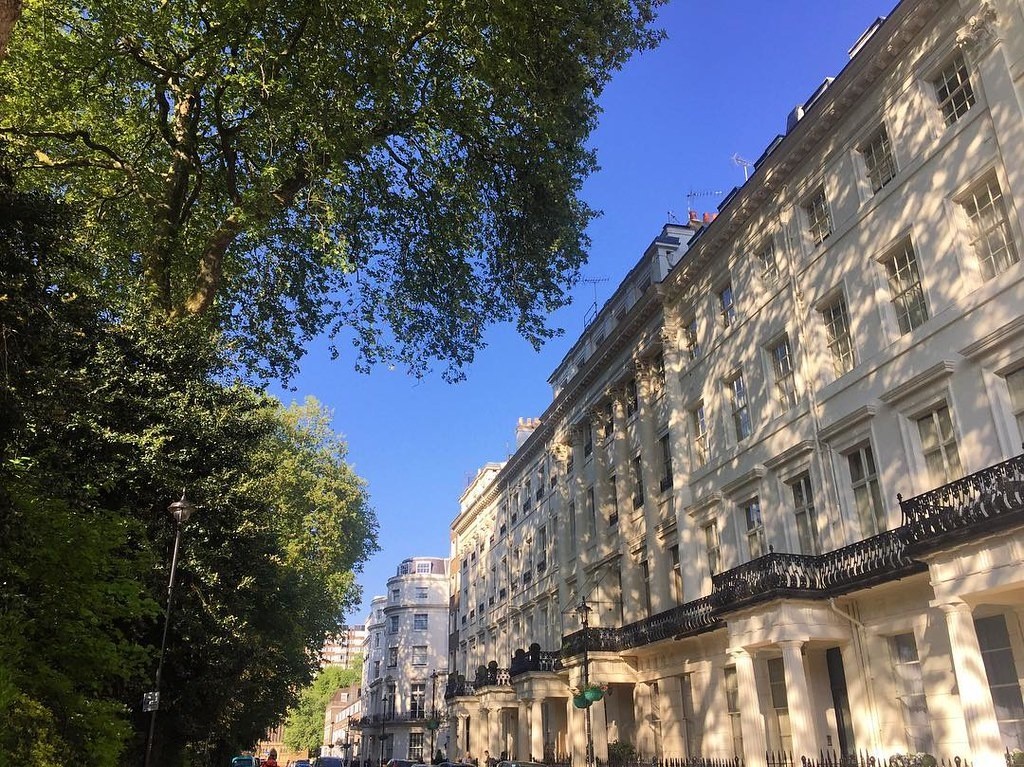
[
  {"left": 139, "top": 485, "right": 197, "bottom": 766},
  {"left": 380, "top": 693, "right": 389, "bottom": 767},
  {"left": 575, "top": 595, "right": 595, "bottom": 767},
  {"left": 429, "top": 669, "right": 438, "bottom": 765},
  {"left": 329, "top": 721, "right": 335, "bottom": 756},
  {"left": 342, "top": 730, "right": 351, "bottom": 767}
]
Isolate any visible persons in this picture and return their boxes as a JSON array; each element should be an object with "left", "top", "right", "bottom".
[
  {"left": 462, "top": 751, "right": 472, "bottom": 764},
  {"left": 483, "top": 750, "right": 490, "bottom": 767}
]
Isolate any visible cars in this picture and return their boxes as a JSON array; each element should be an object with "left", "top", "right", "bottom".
[
  {"left": 315, "top": 756, "right": 343, "bottom": 767},
  {"left": 255, "top": 756, "right": 266, "bottom": 767},
  {"left": 231, "top": 755, "right": 257, "bottom": 767},
  {"left": 294, "top": 761, "right": 312, "bottom": 767},
  {"left": 386, "top": 758, "right": 548, "bottom": 767}
]
